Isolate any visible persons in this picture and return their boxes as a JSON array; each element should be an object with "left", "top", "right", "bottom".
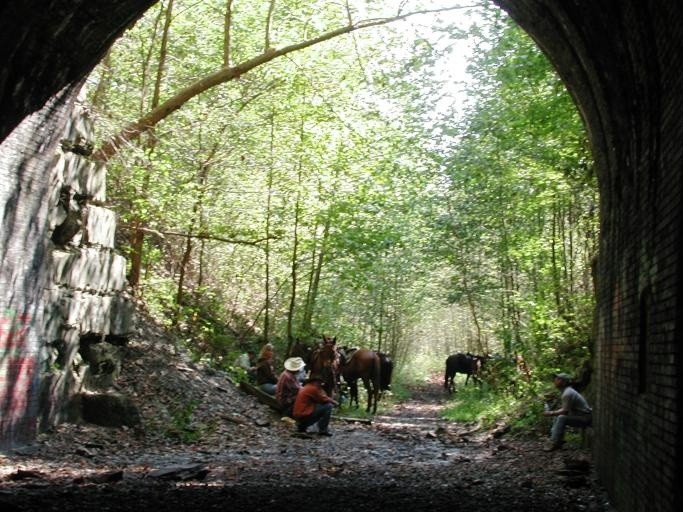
[
  {"left": 256, "top": 344, "right": 277, "bottom": 395},
  {"left": 292, "top": 372, "right": 340, "bottom": 436},
  {"left": 234, "top": 351, "right": 256, "bottom": 373},
  {"left": 276, "top": 356, "right": 307, "bottom": 406},
  {"left": 542, "top": 372, "right": 592, "bottom": 452}
]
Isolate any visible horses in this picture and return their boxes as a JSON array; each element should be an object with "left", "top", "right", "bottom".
[
  {"left": 285, "top": 333, "right": 393, "bottom": 415},
  {"left": 444, "top": 353, "right": 494, "bottom": 396}
]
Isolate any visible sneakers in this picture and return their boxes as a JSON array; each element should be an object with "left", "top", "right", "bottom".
[
  {"left": 542, "top": 444, "right": 559, "bottom": 451},
  {"left": 318, "top": 431, "right": 333, "bottom": 437}
]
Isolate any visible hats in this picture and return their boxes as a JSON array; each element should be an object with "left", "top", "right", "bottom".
[
  {"left": 552, "top": 372, "right": 569, "bottom": 381},
  {"left": 284, "top": 356, "right": 307, "bottom": 371}
]
